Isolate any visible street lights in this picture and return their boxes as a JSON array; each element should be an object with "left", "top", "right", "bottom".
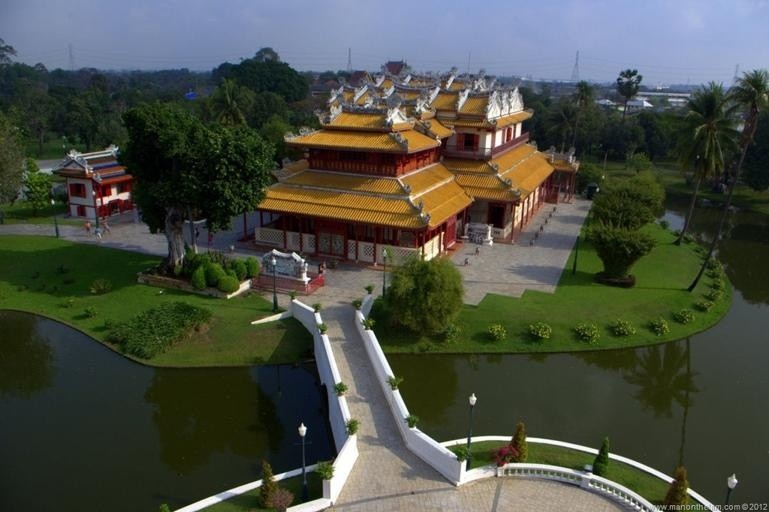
[
  {"left": 298, "top": 421, "right": 308, "bottom": 499},
  {"left": 51, "top": 199, "right": 59, "bottom": 238},
  {"left": 723, "top": 472, "right": 738, "bottom": 512},
  {"left": 382, "top": 249, "right": 387, "bottom": 298},
  {"left": 572, "top": 229, "right": 582, "bottom": 274},
  {"left": 467, "top": 393, "right": 478, "bottom": 471},
  {"left": 271, "top": 257, "right": 279, "bottom": 313},
  {"left": 91, "top": 190, "right": 99, "bottom": 229}
]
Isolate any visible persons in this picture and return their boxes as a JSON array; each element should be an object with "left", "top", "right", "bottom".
[
  {"left": 208, "top": 231, "right": 213, "bottom": 247},
  {"left": 195, "top": 228, "right": 199, "bottom": 245},
  {"left": 85, "top": 216, "right": 110, "bottom": 240},
  {"left": 318, "top": 262, "right": 324, "bottom": 277}
]
